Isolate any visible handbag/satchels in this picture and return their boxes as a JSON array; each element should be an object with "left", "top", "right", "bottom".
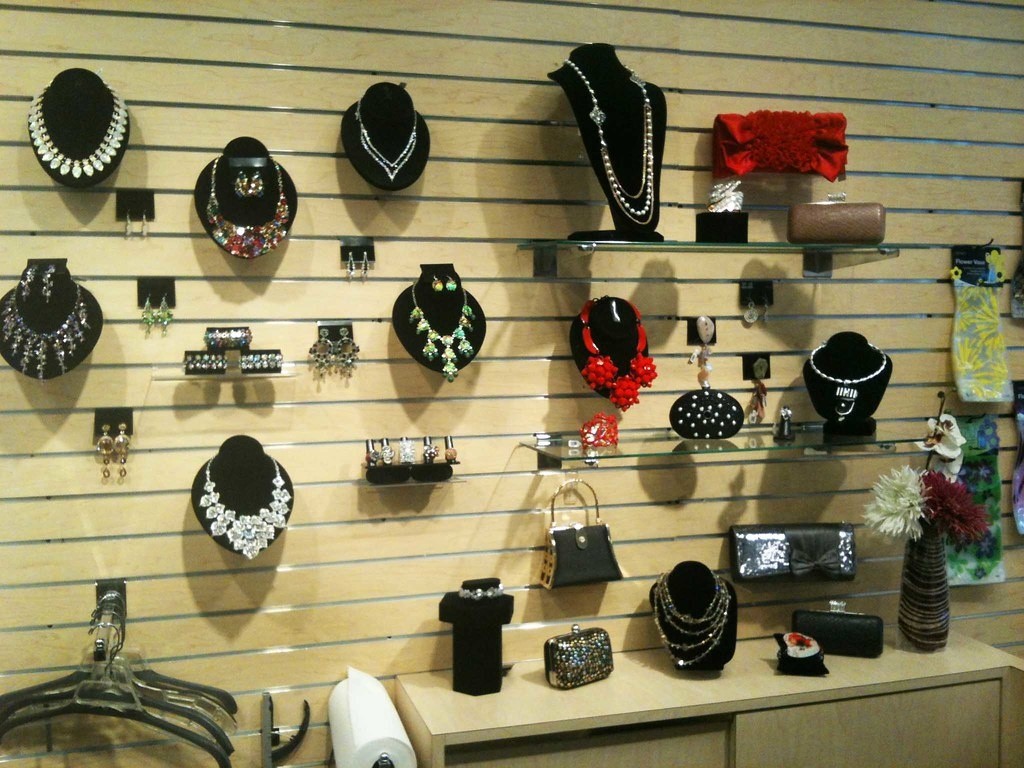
[
  {"left": 539, "top": 478, "right": 624, "bottom": 591},
  {"left": 728, "top": 523, "right": 856, "bottom": 582}
]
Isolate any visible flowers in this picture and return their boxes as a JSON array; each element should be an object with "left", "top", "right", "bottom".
[{"left": 861, "top": 388, "right": 991, "bottom": 545}]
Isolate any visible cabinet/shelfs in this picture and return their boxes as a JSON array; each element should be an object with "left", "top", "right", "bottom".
[
  {"left": 509, "top": 235, "right": 900, "bottom": 469},
  {"left": 395, "top": 627, "right": 1024, "bottom": 768}
]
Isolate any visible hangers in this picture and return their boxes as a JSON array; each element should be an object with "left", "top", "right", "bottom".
[{"left": 0, "top": 587, "right": 237, "bottom": 768}]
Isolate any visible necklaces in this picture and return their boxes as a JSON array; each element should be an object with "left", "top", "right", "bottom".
[
  {"left": 579, "top": 296, "right": 658, "bottom": 412},
  {"left": 810, "top": 344, "right": 887, "bottom": 384},
  {"left": 562, "top": 60, "right": 655, "bottom": 224},
  {"left": 355, "top": 99, "right": 417, "bottom": 182},
  {"left": 206, "top": 156, "right": 289, "bottom": 258},
  {"left": 27, "top": 78, "right": 128, "bottom": 177},
  {"left": 0, "top": 285, "right": 93, "bottom": 380},
  {"left": 409, "top": 279, "right": 475, "bottom": 382},
  {"left": 199, "top": 457, "right": 292, "bottom": 559},
  {"left": 652, "top": 570, "right": 730, "bottom": 665}
]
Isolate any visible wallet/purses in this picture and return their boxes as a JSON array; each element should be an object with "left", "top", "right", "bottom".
[
  {"left": 786, "top": 193, "right": 886, "bottom": 246},
  {"left": 789, "top": 608, "right": 884, "bottom": 659},
  {"left": 545, "top": 624, "right": 615, "bottom": 690}
]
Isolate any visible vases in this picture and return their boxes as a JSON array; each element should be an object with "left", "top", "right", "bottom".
[{"left": 898, "top": 520, "right": 950, "bottom": 650}]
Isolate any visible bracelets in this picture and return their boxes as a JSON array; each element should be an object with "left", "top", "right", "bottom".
[
  {"left": 579, "top": 412, "right": 619, "bottom": 449},
  {"left": 459, "top": 585, "right": 504, "bottom": 600},
  {"left": 708, "top": 179, "right": 743, "bottom": 212}
]
